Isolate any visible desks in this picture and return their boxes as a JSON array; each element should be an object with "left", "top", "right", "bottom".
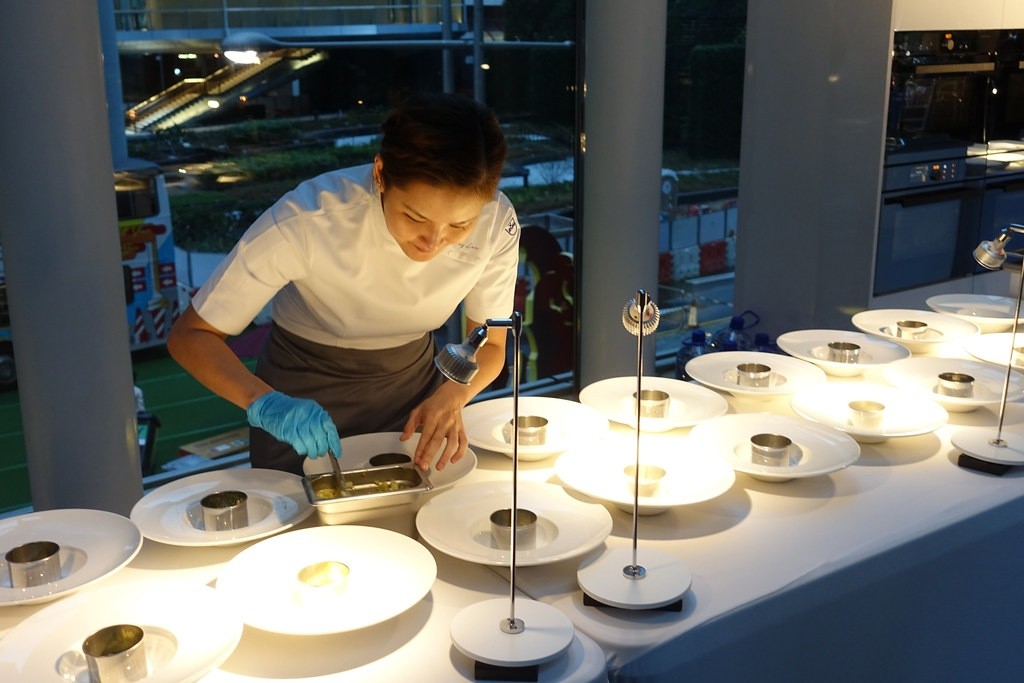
[{"left": 0, "top": 323, "right": 1024, "bottom": 683}]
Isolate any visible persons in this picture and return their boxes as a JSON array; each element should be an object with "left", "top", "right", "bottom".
[{"left": 167, "top": 95, "right": 521, "bottom": 480}]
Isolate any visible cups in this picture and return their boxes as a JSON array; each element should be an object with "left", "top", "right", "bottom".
[
  {"left": 81, "top": 623, "right": 147, "bottom": 683},
  {"left": 1013, "top": 347, "right": 1024, "bottom": 365},
  {"left": 200, "top": 490, "right": 248, "bottom": 531},
  {"left": 296, "top": 319, "right": 976, "bottom": 612},
  {"left": 4, "top": 541, "right": 63, "bottom": 589}
]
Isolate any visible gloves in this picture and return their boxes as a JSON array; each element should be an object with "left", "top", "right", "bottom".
[{"left": 247, "top": 390, "right": 341, "bottom": 460}]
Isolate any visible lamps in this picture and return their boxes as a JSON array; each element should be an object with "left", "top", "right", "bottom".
[
  {"left": 433, "top": 312, "right": 575, "bottom": 666},
  {"left": 576, "top": 288, "right": 693, "bottom": 611},
  {"left": 950, "top": 221, "right": 1024, "bottom": 467}
]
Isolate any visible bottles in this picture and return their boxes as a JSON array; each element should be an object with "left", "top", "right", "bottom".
[{"left": 675, "top": 311, "right": 779, "bottom": 381}]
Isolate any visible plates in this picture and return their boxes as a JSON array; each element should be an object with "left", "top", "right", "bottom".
[
  {"left": 686, "top": 414, "right": 861, "bottom": 484},
  {"left": 685, "top": 351, "right": 828, "bottom": 402},
  {"left": 852, "top": 293, "right": 1024, "bottom": 412},
  {"left": 415, "top": 480, "right": 613, "bottom": 567},
  {"left": 579, "top": 376, "right": 729, "bottom": 433},
  {"left": 461, "top": 397, "right": 610, "bottom": 461},
  {"left": 303, "top": 433, "right": 478, "bottom": 493},
  {"left": 787, "top": 381, "right": 950, "bottom": 443},
  {"left": 178, "top": 167, "right": 234, "bottom": 183},
  {"left": 553, "top": 433, "right": 736, "bottom": 516},
  {"left": 0, "top": 578, "right": 243, "bottom": 683},
  {"left": 776, "top": 329, "right": 912, "bottom": 378},
  {"left": 1, "top": 468, "right": 437, "bottom": 638}
]
